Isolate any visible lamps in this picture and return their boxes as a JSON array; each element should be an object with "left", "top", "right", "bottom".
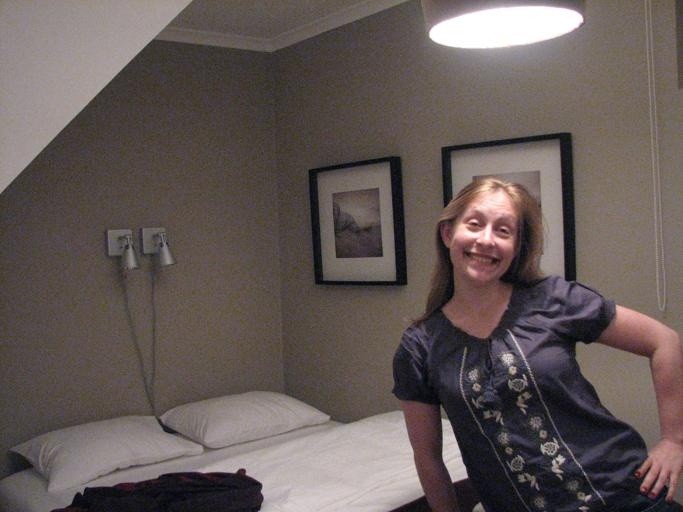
[
  {"left": 118, "top": 234, "right": 141, "bottom": 270},
  {"left": 419, "top": 1, "right": 586, "bottom": 52},
  {"left": 153, "top": 232, "right": 178, "bottom": 267}
]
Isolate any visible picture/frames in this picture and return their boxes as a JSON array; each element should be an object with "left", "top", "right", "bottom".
[
  {"left": 307, "top": 155, "right": 408, "bottom": 286},
  {"left": 441, "top": 132, "right": 577, "bottom": 283}
]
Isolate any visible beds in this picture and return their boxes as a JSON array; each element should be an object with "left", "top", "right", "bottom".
[{"left": 0, "top": 408, "right": 483, "bottom": 512}]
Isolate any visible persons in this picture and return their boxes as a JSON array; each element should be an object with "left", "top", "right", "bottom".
[{"left": 389, "top": 176, "right": 682, "bottom": 512}]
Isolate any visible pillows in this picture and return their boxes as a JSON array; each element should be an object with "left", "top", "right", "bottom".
[
  {"left": 8, "top": 414, "right": 205, "bottom": 495},
  {"left": 158, "top": 390, "right": 332, "bottom": 450}
]
